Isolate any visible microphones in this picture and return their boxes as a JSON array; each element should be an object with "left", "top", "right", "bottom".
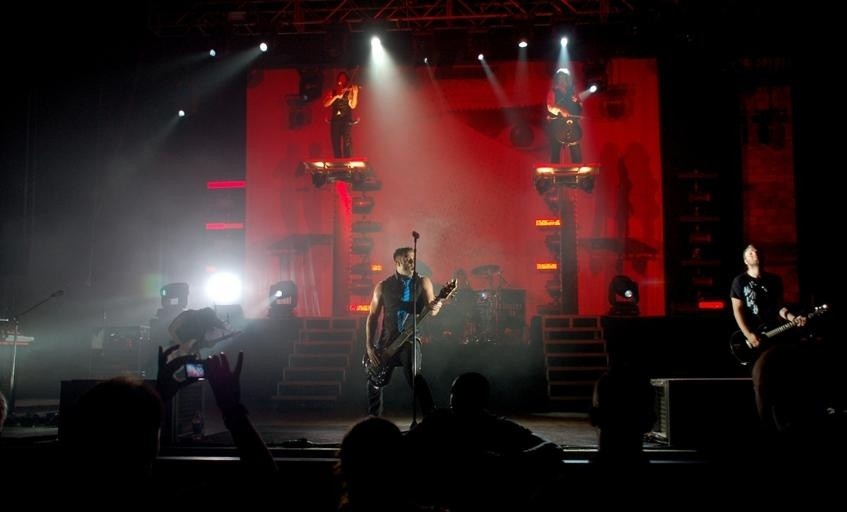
[
  {"left": 51, "top": 290, "right": 65, "bottom": 296},
  {"left": 412, "top": 231, "right": 421, "bottom": 239}
]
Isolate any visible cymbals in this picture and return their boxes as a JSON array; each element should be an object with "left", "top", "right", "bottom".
[
  {"left": 415, "top": 260, "right": 432, "bottom": 277},
  {"left": 472, "top": 264, "right": 501, "bottom": 279}
]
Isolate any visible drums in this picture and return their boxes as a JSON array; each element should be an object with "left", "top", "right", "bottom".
[
  {"left": 505, "top": 304, "right": 525, "bottom": 328},
  {"left": 476, "top": 292, "right": 498, "bottom": 311}
]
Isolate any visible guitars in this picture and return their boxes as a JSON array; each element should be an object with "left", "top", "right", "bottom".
[
  {"left": 363, "top": 278, "right": 461, "bottom": 389},
  {"left": 164, "top": 330, "right": 239, "bottom": 376},
  {"left": 730, "top": 304, "right": 829, "bottom": 363}
]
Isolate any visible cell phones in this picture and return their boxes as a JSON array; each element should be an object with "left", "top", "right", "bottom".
[{"left": 183, "top": 357, "right": 207, "bottom": 382}]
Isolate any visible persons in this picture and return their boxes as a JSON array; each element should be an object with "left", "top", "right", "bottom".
[
  {"left": 731, "top": 244, "right": 807, "bottom": 348},
  {"left": 547, "top": 67, "right": 584, "bottom": 163},
  {"left": 321, "top": 71, "right": 359, "bottom": 158},
  {"left": 365, "top": 247, "right": 443, "bottom": 416}
]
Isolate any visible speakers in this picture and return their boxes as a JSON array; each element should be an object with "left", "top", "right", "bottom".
[
  {"left": 57, "top": 376, "right": 206, "bottom": 446},
  {"left": 649, "top": 376, "right": 755, "bottom": 450}
]
